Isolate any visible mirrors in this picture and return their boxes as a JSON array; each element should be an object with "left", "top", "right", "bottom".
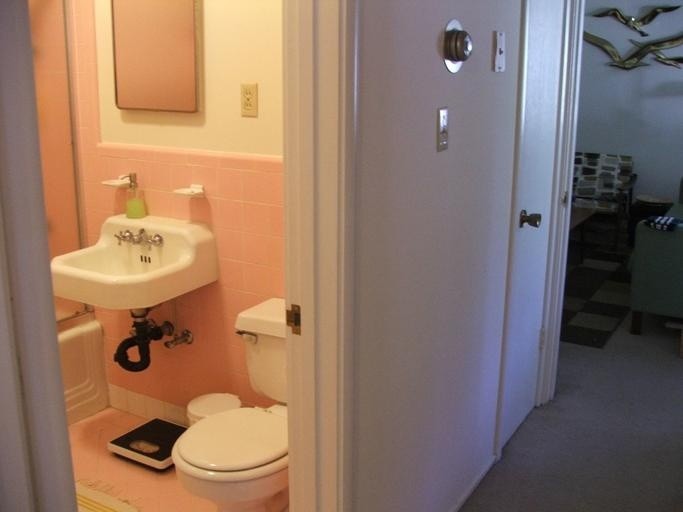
[{"left": 109, "top": 1, "right": 202, "bottom": 115}]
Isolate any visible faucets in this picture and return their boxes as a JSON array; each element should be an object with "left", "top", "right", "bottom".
[{"left": 129, "top": 226, "right": 147, "bottom": 247}]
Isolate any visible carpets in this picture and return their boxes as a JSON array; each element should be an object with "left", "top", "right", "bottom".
[
  {"left": 559, "top": 241, "right": 631, "bottom": 349},
  {"left": 73, "top": 471, "right": 145, "bottom": 512}
]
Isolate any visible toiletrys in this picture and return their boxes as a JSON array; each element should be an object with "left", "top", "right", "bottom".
[{"left": 120, "top": 173, "right": 146, "bottom": 219}]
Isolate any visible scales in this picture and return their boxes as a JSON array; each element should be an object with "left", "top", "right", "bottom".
[{"left": 106, "top": 416, "right": 189, "bottom": 469}]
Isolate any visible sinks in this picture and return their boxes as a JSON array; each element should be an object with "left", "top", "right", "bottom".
[{"left": 50, "top": 214, "right": 217, "bottom": 310}]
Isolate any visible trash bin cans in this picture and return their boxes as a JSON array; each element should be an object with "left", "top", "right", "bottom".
[{"left": 186, "top": 392, "right": 242, "bottom": 429}]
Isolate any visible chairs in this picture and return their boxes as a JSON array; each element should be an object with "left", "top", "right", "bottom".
[{"left": 629, "top": 175, "right": 683, "bottom": 336}]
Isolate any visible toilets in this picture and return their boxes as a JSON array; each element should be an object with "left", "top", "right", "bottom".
[{"left": 171, "top": 296, "right": 288, "bottom": 512}]
